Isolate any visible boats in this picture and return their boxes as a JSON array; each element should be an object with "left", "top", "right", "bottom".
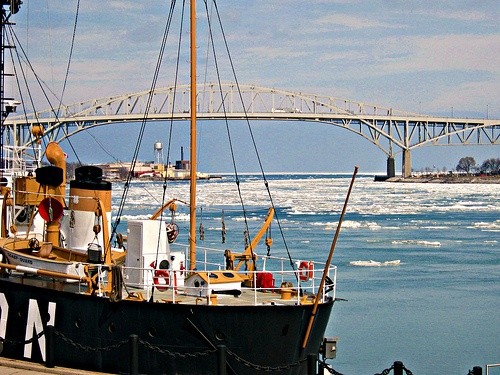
[
  {"left": 85, "top": 146, "right": 223, "bottom": 181},
  {"left": 0, "top": 1, "right": 359, "bottom": 375}
]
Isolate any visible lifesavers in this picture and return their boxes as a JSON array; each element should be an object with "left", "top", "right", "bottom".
[
  {"left": 153, "top": 270, "right": 171, "bottom": 292},
  {"left": 299, "top": 262, "right": 313, "bottom": 280}
]
